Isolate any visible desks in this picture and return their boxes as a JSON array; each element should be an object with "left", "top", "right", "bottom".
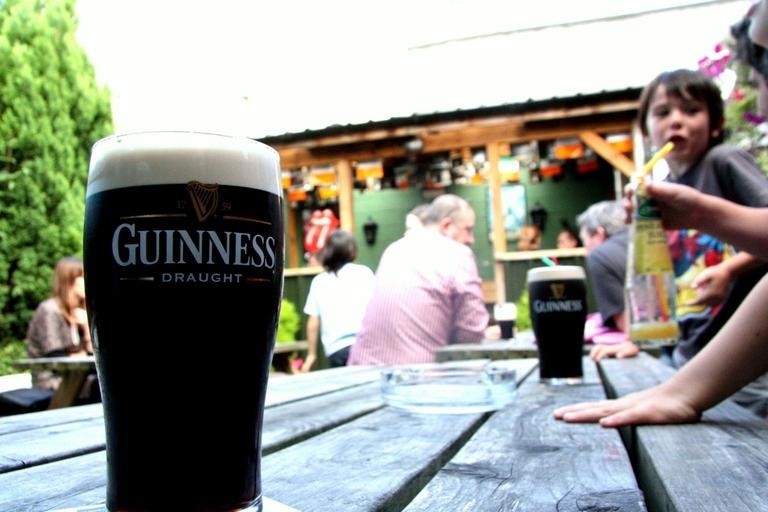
[
  {"left": 433, "top": 331, "right": 612, "bottom": 366},
  {"left": 8, "top": 339, "right": 311, "bottom": 410},
  {"left": 0, "top": 364, "right": 768, "bottom": 510}
]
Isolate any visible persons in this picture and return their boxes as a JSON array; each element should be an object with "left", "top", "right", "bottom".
[
  {"left": 558, "top": 201, "right": 629, "bottom": 332},
  {"left": 300, "top": 194, "right": 490, "bottom": 373},
  {"left": 553, "top": 0, "right": 768, "bottom": 427},
  {"left": 27, "top": 256, "right": 102, "bottom": 399},
  {"left": 639, "top": 68, "right": 768, "bottom": 365}
]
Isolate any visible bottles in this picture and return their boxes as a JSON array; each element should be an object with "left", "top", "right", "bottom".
[{"left": 625, "top": 170, "right": 680, "bottom": 348}]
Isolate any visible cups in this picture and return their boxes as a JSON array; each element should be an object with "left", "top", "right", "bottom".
[
  {"left": 494, "top": 304, "right": 517, "bottom": 338},
  {"left": 82, "top": 130, "right": 286, "bottom": 512},
  {"left": 527, "top": 265, "right": 589, "bottom": 386}
]
